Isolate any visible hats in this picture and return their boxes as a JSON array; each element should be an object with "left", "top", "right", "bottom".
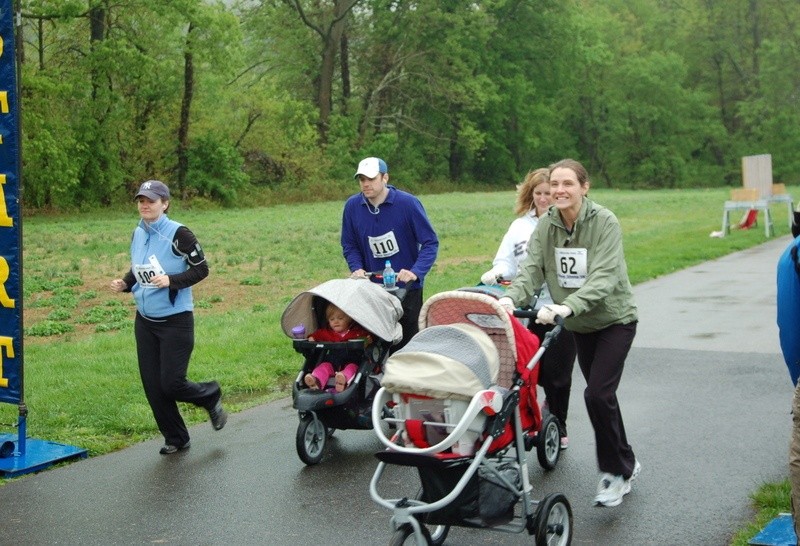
[
  {"left": 134, "top": 180, "right": 169, "bottom": 202},
  {"left": 354, "top": 157, "right": 387, "bottom": 179}
]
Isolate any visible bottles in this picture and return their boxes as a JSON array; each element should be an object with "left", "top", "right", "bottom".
[
  {"left": 383, "top": 260, "right": 396, "bottom": 289},
  {"left": 291, "top": 323, "right": 305, "bottom": 339}
]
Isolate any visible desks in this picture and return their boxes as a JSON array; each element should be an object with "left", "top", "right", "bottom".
[{"left": 723, "top": 194, "right": 794, "bottom": 238}]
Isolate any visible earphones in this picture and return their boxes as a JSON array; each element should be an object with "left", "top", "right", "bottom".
[{"left": 163, "top": 204, "right": 166, "bottom": 209}]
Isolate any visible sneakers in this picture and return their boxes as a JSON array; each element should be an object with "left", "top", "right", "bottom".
[
  {"left": 560, "top": 437, "right": 567, "bottom": 449},
  {"left": 160, "top": 438, "right": 190, "bottom": 454},
  {"left": 594, "top": 459, "right": 641, "bottom": 507},
  {"left": 207, "top": 380, "right": 227, "bottom": 430}
]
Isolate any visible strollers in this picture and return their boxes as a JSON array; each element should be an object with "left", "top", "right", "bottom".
[
  {"left": 369, "top": 277, "right": 573, "bottom": 546},
  {"left": 281, "top": 272, "right": 416, "bottom": 466}
]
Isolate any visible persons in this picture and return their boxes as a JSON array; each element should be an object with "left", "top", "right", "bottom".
[
  {"left": 304, "top": 303, "right": 372, "bottom": 393},
  {"left": 498, "top": 159, "right": 640, "bottom": 507},
  {"left": 340, "top": 157, "right": 439, "bottom": 418},
  {"left": 777, "top": 231, "right": 800, "bottom": 546},
  {"left": 481, "top": 168, "right": 577, "bottom": 449},
  {"left": 110, "top": 180, "right": 228, "bottom": 456}
]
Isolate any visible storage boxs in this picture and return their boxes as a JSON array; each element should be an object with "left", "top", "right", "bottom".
[
  {"left": 773, "top": 184, "right": 785, "bottom": 196},
  {"left": 730, "top": 188, "right": 759, "bottom": 202}
]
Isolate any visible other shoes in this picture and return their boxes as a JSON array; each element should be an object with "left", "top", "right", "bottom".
[
  {"left": 304, "top": 373, "right": 320, "bottom": 391},
  {"left": 335, "top": 373, "right": 346, "bottom": 393}
]
